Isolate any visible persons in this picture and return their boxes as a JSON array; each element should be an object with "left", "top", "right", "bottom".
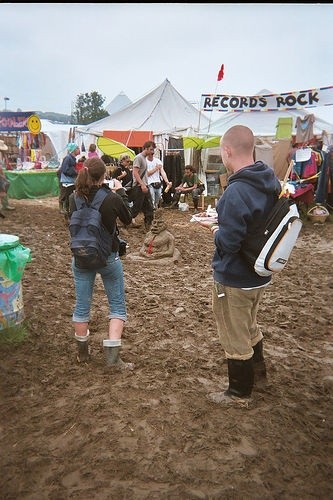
[
  {"left": 127, "top": 218, "right": 181, "bottom": 266},
  {"left": 57, "top": 142, "right": 132, "bottom": 221},
  {"left": 199, "top": 125, "right": 285, "bottom": 409},
  {"left": 165, "top": 165, "right": 205, "bottom": 214},
  {"left": 0, "top": 140, "right": 17, "bottom": 219},
  {"left": 70, "top": 156, "right": 133, "bottom": 371},
  {"left": 145, "top": 149, "right": 170, "bottom": 220},
  {"left": 128, "top": 142, "right": 153, "bottom": 232}
]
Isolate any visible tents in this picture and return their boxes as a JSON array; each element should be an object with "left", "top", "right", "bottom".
[{"left": 0, "top": 78, "right": 333, "bottom": 174}]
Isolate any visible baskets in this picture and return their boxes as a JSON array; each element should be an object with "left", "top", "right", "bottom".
[{"left": 307, "top": 206, "right": 329, "bottom": 223}]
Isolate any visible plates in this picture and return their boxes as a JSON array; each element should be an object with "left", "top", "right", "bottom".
[{"left": 193, "top": 212, "right": 218, "bottom": 221}]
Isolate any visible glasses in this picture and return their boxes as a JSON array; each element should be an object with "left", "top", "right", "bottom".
[{"left": 124, "top": 159, "right": 131, "bottom": 163}]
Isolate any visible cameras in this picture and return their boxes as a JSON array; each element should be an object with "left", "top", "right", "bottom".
[{"left": 104, "top": 180, "right": 114, "bottom": 188}]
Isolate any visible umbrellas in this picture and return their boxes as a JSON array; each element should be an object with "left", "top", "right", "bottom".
[
  {"left": 98, "top": 137, "right": 136, "bottom": 160},
  {"left": 180, "top": 136, "right": 205, "bottom": 149},
  {"left": 196, "top": 136, "right": 223, "bottom": 150}
]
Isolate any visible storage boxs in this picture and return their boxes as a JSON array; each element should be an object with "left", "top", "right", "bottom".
[
  {"left": 180, "top": 194, "right": 205, "bottom": 210},
  {"left": 205, "top": 163, "right": 225, "bottom": 196}
]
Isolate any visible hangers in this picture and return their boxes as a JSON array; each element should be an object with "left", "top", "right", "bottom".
[{"left": 165, "top": 148, "right": 181, "bottom": 155}]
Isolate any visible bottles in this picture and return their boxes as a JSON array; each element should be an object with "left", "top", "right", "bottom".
[{"left": 164, "top": 182, "right": 172, "bottom": 193}]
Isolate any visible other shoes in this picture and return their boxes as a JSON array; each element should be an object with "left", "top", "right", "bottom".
[{"left": 126, "top": 223, "right": 142, "bottom": 228}]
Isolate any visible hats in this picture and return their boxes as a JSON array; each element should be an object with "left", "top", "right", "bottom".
[
  {"left": 65, "top": 143, "right": 78, "bottom": 153},
  {"left": 0, "top": 140, "right": 8, "bottom": 150}
]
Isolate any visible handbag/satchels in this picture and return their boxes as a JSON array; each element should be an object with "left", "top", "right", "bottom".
[
  {"left": 151, "top": 183, "right": 162, "bottom": 189},
  {"left": 117, "top": 239, "right": 126, "bottom": 256},
  {"left": 124, "top": 186, "right": 133, "bottom": 203}
]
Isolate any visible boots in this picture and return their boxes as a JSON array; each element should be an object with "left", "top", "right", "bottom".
[
  {"left": 62, "top": 201, "right": 69, "bottom": 212},
  {"left": 165, "top": 199, "right": 178, "bottom": 209},
  {"left": 207, "top": 359, "right": 252, "bottom": 408},
  {"left": 59, "top": 202, "right": 65, "bottom": 213},
  {"left": 251, "top": 338, "right": 266, "bottom": 382},
  {"left": 75, "top": 329, "right": 91, "bottom": 363},
  {"left": 103, "top": 339, "right": 136, "bottom": 372},
  {"left": 193, "top": 200, "right": 198, "bottom": 214},
  {"left": 2, "top": 196, "right": 15, "bottom": 210}
]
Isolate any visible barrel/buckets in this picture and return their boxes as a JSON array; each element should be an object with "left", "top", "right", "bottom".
[{"left": 0, "top": 234, "right": 24, "bottom": 348}]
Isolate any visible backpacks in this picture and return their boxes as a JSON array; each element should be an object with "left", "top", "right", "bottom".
[
  {"left": 104, "top": 164, "right": 118, "bottom": 180},
  {"left": 69, "top": 208, "right": 113, "bottom": 268},
  {"left": 243, "top": 198, "right": 304, "bottom": 277}
]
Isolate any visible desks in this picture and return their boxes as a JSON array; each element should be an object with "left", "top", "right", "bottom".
[
  {"left": 4, "top": 170, "right": 60, "bottom": 199},
  {"left": 289, "top": 183, "right": 314, "bottom": 220}
]
